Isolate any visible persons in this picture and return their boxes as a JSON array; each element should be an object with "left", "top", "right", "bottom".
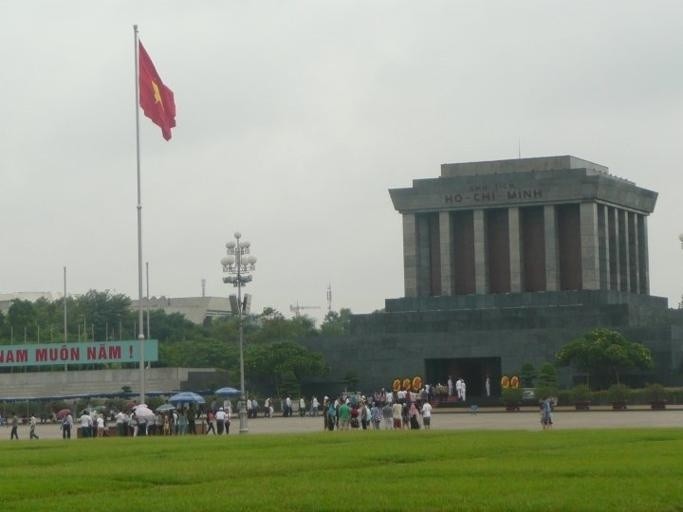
[
  {"left": 460, "top": 379, "right": 465, "bottom": 402},
  {"left": 55, "top": 403, "right": 233, "bottom": 440},
  {"left": 455, "top": 377, "right": 462, "bottom": 400},
  {"left": 7, "top": 413, "right": 19, "bottom": 440},
  {"left": 29, "top": 414, "right": 39, "bottom": 439},
  {"left": 541, "top": 398, "right": 553, "bottom": 429},
  {"left": 246, "top": 393, "right": 320, "bottom": 418},
  {"left": 323, "top": 383, "right": 447, "bottom": 432},
  {"left": 235, "top": 397, "right": 240, "bottom": 418},
  {"left": 484, "top": 377, "right": 490, "bottom": 396},
  {"left": 447, "top": 376, "right": 452, "bottom": 395}
]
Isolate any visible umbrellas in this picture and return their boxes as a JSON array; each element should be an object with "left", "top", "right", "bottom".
[
  {"left": 168, "top": 391, "right": 205, "bottom": 409},
  {"left": 214, "top": 387, "right": 241, "bottom": 401}
]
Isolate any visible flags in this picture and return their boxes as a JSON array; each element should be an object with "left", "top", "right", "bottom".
[{"left": 138, "top": 42, "right": 178, "bottom": 142}]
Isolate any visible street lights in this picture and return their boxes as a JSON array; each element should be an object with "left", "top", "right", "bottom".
[{"left": 219, "top": 231, "right": 258, "bottom": 433}]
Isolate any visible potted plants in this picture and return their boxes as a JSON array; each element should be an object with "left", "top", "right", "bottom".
[{"left": 502, "top": 382, "right": 672, "bottom": 412}]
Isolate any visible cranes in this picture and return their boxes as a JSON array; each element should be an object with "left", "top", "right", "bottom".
[{"left": 289, "top": 299, "right": 323, "bottom": 318}]
